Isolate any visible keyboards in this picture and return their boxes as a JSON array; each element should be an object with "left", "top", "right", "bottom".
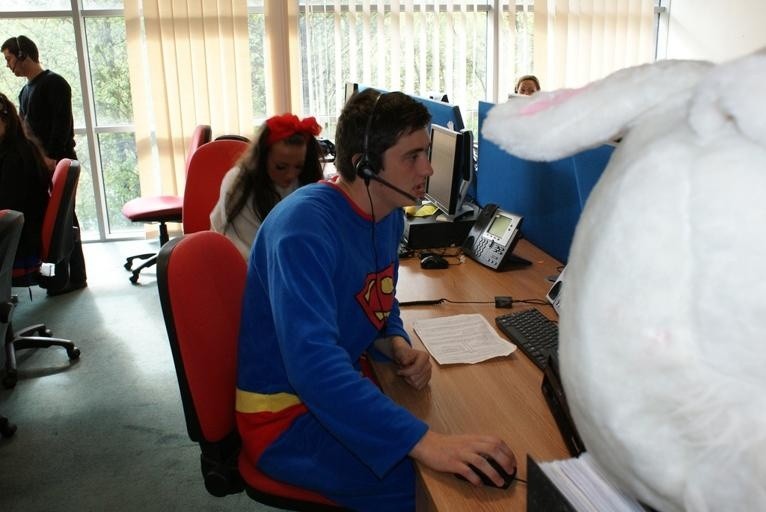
[{"left": 495, "top": 306, "right": 558, "bottom": 371}]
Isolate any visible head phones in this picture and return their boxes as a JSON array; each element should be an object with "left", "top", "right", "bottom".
[
  {"left": 356, "top": 91, "right": 379, "bottom": 178},
  {"left": 16, "top": 37, "right": 27, "bottom": 61},
  {"left": 515, "top": 76, "right": 541, "bottom": 93}
]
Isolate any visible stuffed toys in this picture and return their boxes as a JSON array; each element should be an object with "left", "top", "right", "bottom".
[{"left": 481, "top": 42, "right": 766, "bottom": 512}]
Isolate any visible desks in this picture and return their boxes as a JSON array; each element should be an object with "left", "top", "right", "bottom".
[{"left": 316, "top": 152, "right": 567, "bottom": 512}]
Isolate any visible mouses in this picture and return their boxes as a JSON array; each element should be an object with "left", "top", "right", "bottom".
[
  {"left": 454, "top": 454, "right": 518, "bottom": 490},
  {"left": 420, "top": 256, "right": 448, "bottom": 269}
]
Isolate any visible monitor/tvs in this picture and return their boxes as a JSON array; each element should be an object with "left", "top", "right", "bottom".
[
  {"left": 431, "top": 93, "right": 448, "bottom": 102},
  {"left": 424, "top": 123, "right": 475, "bottom": 222},
  {"left": 344, "top": 81, "right": 358, "bottom": 104}
]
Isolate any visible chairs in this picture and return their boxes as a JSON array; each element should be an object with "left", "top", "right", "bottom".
[
  {"left": 120, "top": 124, "right": 212, "bottom": 283},
  {"left": 151, "top": 231, "right": 334, "bottom": 512},
  {"left": 0, "top": 156, "right": 82, "bottom": 387},
  {"left": 182, "top": 138, "right": 250, "bottom": 233},
  {"left": 0, "top": 209, "right": 25, "bottom": 438}
]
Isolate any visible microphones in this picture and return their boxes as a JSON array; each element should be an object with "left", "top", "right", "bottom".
[
  {"left": 363, "top": 168, "right": 422, "bottom": 207},
  {"left": 12, "top": 58, "right": 20, "bottom": 73}
]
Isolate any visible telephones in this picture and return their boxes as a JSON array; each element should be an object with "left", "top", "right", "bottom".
[{"left": 460, "top": 204, "right": 524, "bottom": 273}]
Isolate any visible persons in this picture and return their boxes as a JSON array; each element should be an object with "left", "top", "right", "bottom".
[
  {"left": 234, "top": 87, "right": 518, "bottom": 512},
  {"left": 1, "top": 35, "right": 88, "bottom": 297},
  {"left": 0, "top": 91, "right": 53, "bottom": 265},
  {"left": 209, "top": 112, "right": 326, "bottom": 264},
  {"left": 515, "top": 75, "right": 541, "bottom": 95}
]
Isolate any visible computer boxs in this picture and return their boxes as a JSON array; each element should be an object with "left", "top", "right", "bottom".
[
  {"left": 541, "top": 350, "right": 586, "bottom": 458},
  {"left": 401, "top": 198, "right": 484, "bottom": 250}
]
